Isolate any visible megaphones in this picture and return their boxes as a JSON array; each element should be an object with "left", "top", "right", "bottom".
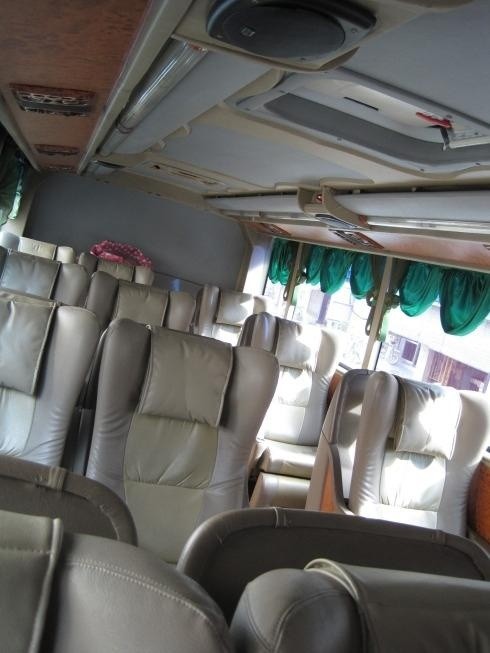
[
  {"left": 297, "top": 186, "right": 369, "bottom": 229},
  {"left": 172, "top": 0, "right": 376, "bottom": 72}
]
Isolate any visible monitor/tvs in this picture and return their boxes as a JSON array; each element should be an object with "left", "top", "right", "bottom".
[{"left": 399, "top": 339, "right": 420, "bottom": 366}]
